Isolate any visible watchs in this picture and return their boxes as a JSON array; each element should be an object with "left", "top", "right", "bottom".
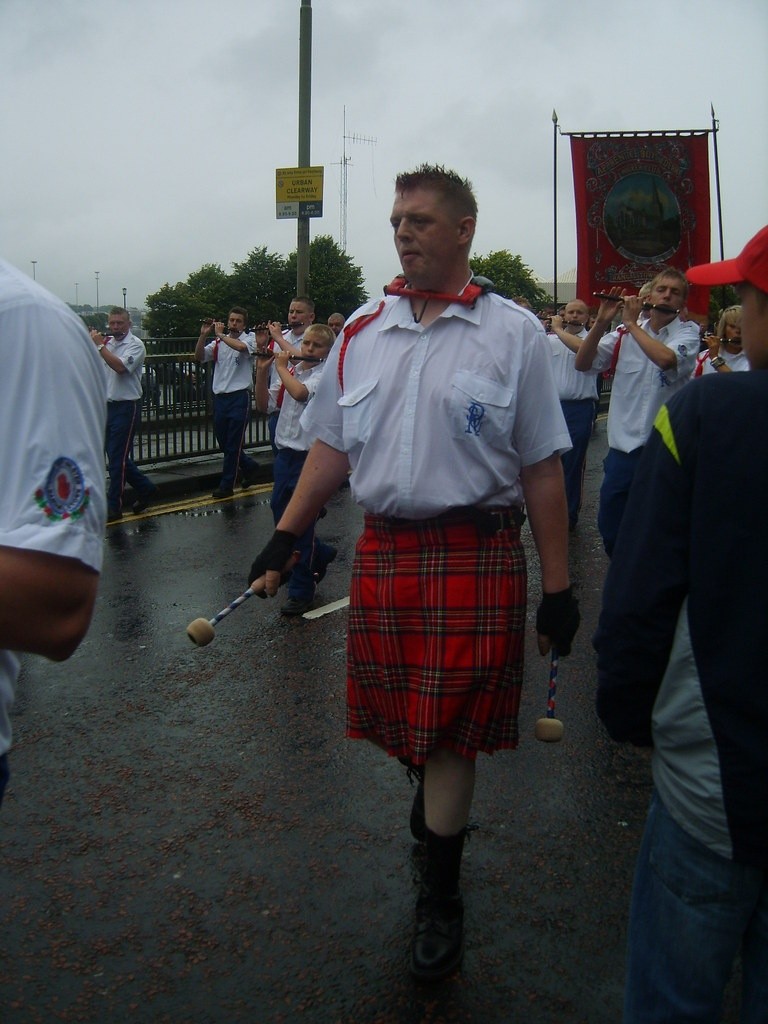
[{"left": 97, "top": 344, "right": 105, "bottom": 350}]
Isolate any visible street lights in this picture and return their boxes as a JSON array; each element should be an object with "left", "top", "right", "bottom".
[
  {"left": 75, "top": 282, "right": 79, "bottom": 313},
  {"left": 95, "top": 271, "right": 100, "bottom": 313},
  {"left": 122, "top": 287, "right": 127, "bottom": 309},
  {"left": 31, "top": 260, "right": 38, "bottom": 280}
]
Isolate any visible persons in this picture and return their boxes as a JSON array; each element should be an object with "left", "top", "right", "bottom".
[
  {"left": 88, "top": 306, "right": 160, "bottom": 521},
  {"left": 0, "top": 258, "right": 109, "bottom": 804},
  {"left": 196, "top": 161, "right": 768, "bottom": 1024}
]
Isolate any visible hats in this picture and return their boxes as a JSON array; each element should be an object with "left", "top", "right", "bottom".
[{"left": 686, "top": 226, "right": 768, "bottom": 292}]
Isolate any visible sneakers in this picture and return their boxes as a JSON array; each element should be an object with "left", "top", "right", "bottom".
[
  {"left": 311, "top": 546, "right": 337, "bottom": 584},
  {"left": 281, "top": 596, "right": 314, "bottom": 616}
]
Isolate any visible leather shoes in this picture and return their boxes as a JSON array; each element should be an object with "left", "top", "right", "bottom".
[
  {"left": 410, "top": 778, "right": 426, "bottom": 840},
  {"left": 410, "top": 885, "right": 464, "bottom": 983}
]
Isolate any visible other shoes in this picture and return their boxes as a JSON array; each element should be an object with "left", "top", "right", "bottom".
[
  {"left": 131, "top": 486, "right": 162, "bottom": 515},
  {"left": 241, "top": 470, "right": 261, "bottom": 488},
  {"left": 212, "top": 487, "right": 235, "bottom": 498}
]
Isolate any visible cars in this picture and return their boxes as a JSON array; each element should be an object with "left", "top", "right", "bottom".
[
  {"left": 141, "top": 364, "right": 162, "bottom": 407},
  {"left": 166, "top": 361, "right": 206, "bottom": 386}
]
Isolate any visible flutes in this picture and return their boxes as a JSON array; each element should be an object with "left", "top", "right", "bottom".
[
  {"left": 251, "top": 350, "right": 326, "bottom": 362},
  {"left": 199, "top": 319, "right": 244, "bottom": 334},
  {"left": 699, "top": 337, "right": 743, "bottom": 344},
  {"left": 245, "top": 322, "right": 304, "bottom": 332},
  {"left": 592, "top": 290, "right": 681, "bottom": 314},
  {"left": 101, "top": 332, "right": 126, "bottom": 335},
  {"left": 536, "top": 315, "right": 584, "bottom": 326}
]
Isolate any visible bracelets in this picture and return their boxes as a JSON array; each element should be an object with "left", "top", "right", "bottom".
[
  {"left": 221, "top": 334, "right": 226, "bottom": 339},
  {"left": 710, "top": 357, "right": 725, "bottom": 368}
]
